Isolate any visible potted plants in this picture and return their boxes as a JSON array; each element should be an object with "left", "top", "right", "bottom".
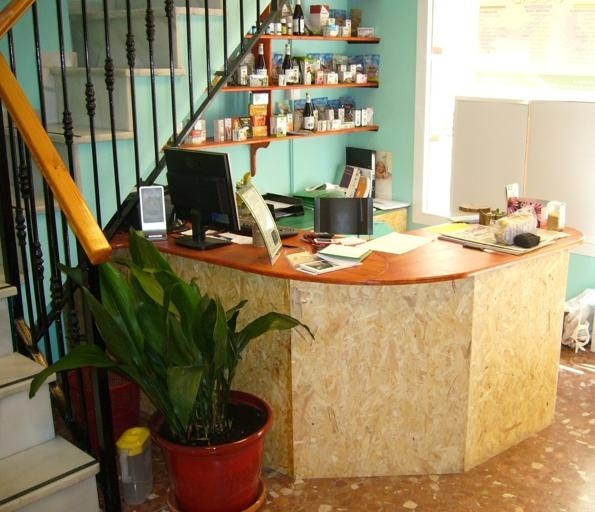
[{"left": 24, "top": 225, "right": 318, "bottom": 511}]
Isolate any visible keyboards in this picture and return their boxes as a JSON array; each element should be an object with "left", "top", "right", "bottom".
[{"left": 238, "top": 219, "right": 298, "bottom": 239}]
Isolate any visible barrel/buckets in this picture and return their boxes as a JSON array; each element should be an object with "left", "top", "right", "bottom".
[{"left": 115, "top": 426, "right": 155, "bottom": 507}]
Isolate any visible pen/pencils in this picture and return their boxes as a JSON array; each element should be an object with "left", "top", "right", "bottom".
[{"left": 282, "top": 244, "right": 298, "bottom": 248}]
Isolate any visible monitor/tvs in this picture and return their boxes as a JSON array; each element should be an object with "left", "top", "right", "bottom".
[{"left": 164, "top": 147, "right": 241, "bottom": 251}]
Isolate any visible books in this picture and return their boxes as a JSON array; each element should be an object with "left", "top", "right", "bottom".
[
  {"left": 319, "top": 244, "right": 373, "bottom": 262},
  {"left": 436, "top": 221, "right": 571, "bottom": 256},
  {"left": 341, "top": 164, "right": 373, "bottom": 199},
  {"left": 297, "top": 258, "right": 363, "bottom": 276}
]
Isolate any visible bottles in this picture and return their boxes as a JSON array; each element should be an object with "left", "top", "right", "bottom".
[
  {"left": 214, "top": 120, "right": 224, "bottom": 142},
  {"left": 266, "top": 16, "right": 292, "bottom": 35},
  {"left": 255, "top": 43, "right": 267, "bottom": 75},
  {"left": 293, "top": 1, "right": 305, "bottom": 36},
  {"left": 278, "top": 75, "right": 287, "bottom": 87},
  {"left": 282, "top": 44, "right": 294, "bottom": 85},
  {"left": 303, "top": 92, "right": 314, "bottom": 131}
]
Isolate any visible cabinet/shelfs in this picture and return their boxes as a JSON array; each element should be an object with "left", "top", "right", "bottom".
[
  {"left": 372, "top": 207, "right": 408, "bottom": 232},
  {"left": 162, "top": 29, "right": 384, "bottom": 178}
]
